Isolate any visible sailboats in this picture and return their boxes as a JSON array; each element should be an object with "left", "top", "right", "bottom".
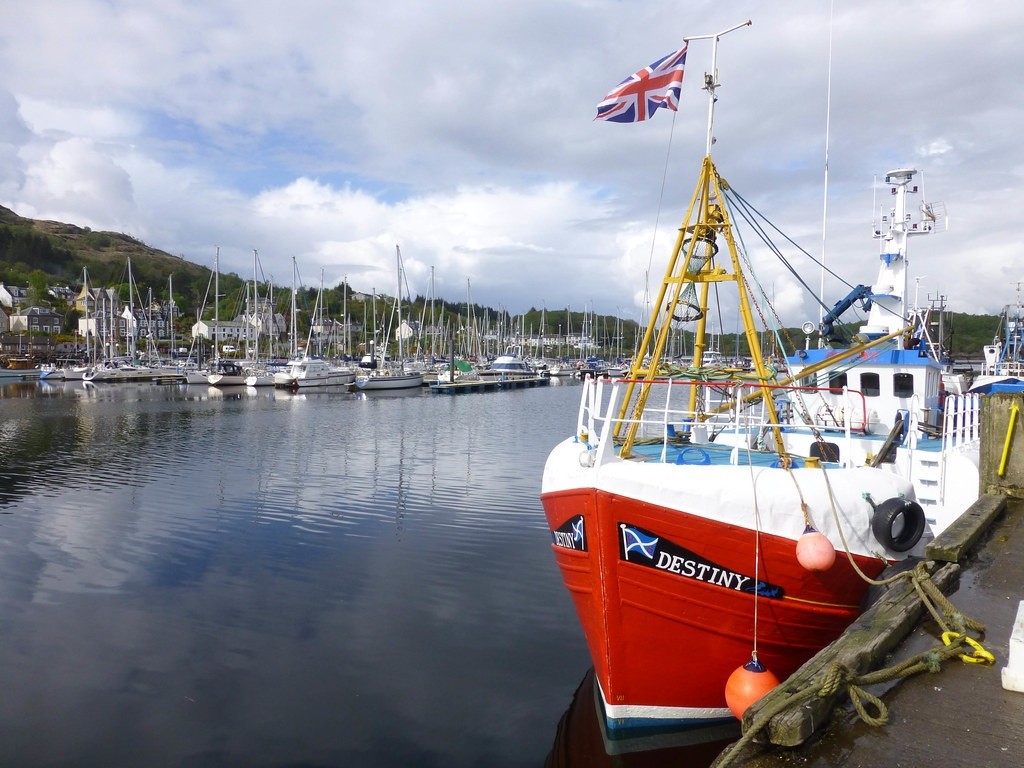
[{"left": 1, "top": 245, "right": 789, "bottom": 392}]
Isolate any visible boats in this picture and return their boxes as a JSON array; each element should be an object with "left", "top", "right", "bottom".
[{"left": 540, "top": 18, "right": 1023, "bottom": 729}]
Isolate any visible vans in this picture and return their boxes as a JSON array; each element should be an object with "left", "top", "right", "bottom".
[
  {"left": 223, "top": 345, "right": 235, "bottom": 354},
  {"left": 248, "top": 347, "right": 254, "bottom": 353}
]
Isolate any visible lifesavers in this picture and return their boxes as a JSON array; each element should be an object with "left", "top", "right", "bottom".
[{"left": 871, "top": 497, "right": 926, "bottom": 552}]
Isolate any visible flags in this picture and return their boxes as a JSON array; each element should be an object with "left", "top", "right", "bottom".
[{"left": 592, "top": 42, "right": 687, "bottom": 123}]
